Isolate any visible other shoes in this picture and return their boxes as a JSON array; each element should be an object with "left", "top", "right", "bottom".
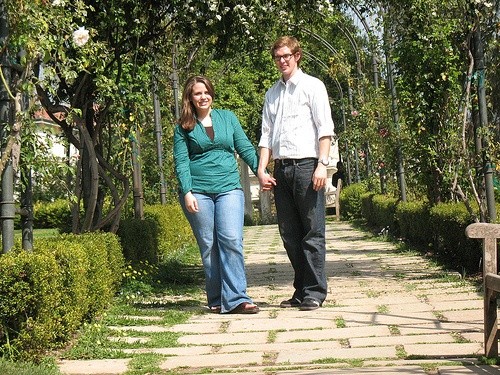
[
  {"left": 280, "top": 297, "right": 302, "bottom": 307},
  {"left": 210, "top": 306, "right": 221, "bottom": 314},
  {"left": 229, "top": 302, "right": 259, "bottom": 314},
  {"left": 299, "top": 298, "right": 320, "bottom": 311}
]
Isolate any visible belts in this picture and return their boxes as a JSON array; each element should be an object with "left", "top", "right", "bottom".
[{"left": 275, "top": 158, "right": 314, "bottom": 167}]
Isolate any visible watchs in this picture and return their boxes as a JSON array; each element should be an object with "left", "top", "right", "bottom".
[{"left": 318, "top": 159, "right": 329, "bottom": 166}]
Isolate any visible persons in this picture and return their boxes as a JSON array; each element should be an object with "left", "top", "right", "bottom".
[
  {"left": 173, "top": 75, "right": 276, "bottom": 313},
  {"left": 257, "top": 36, "right": 336, "bottom": 310}
]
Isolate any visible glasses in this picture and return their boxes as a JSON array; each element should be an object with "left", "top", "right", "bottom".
[{"left": 274, "top": 52, "right": 295, "bottom": 61}]
